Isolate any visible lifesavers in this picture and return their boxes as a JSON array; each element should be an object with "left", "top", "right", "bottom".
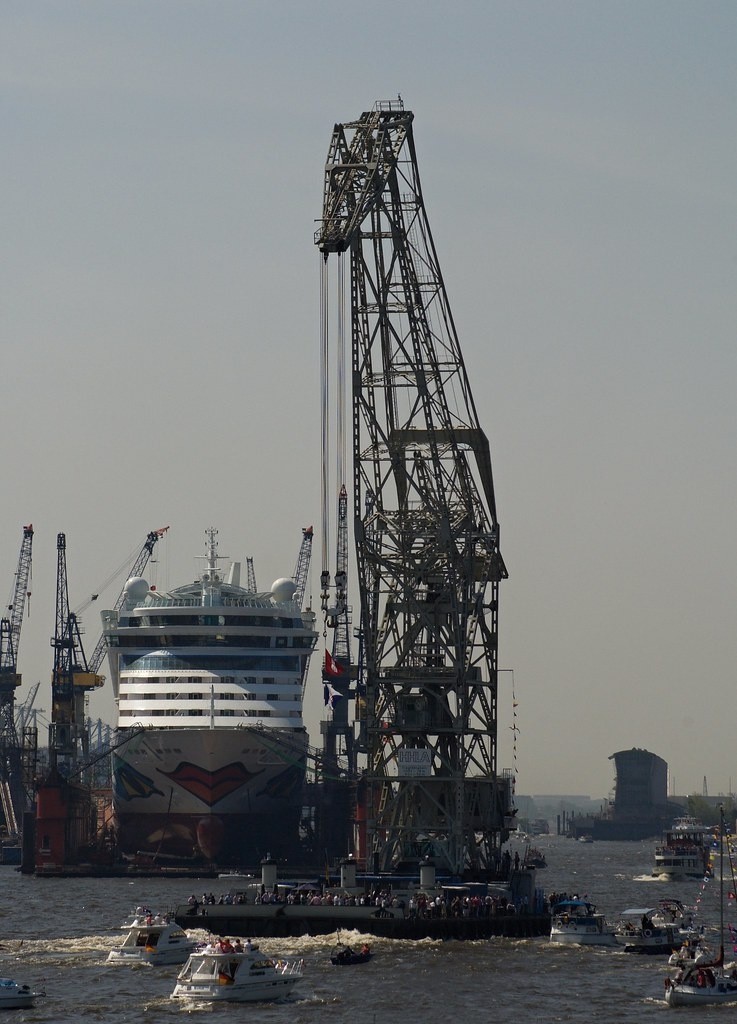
[
  {"left": 556, "top": 920, "right": 563, "bottom": 928},
  {"left": 645, "top": 929, "right": 652, "bottom": 937},
  {"left": 697, "top": 975, "right": 703, "bottom": 986}
]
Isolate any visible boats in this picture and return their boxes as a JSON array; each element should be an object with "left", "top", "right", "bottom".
[
  {"left": 550, "top": 897, "right": 617, "bottom": 945},
  {"left": 616, "top": 907, "right": 706, "bottom": 952},
  {"left": 0, "top": 977, "right": 44, "bottom": 1009},
  {"left": 655, "top": 897, "right": 695, "bottom": 930},
  {"left": 577, "top": 834, "right": 593, "bottom": 844},
  {"left": 329, "top": 943, "right": 376, "bottom": 965},
  {"left": 653, "top": 810, "right": 716, "bottom": 881},
  {"left": 666, "top": 802, "right": 737, "bottom": 1007},
  {"left": 169, "top": 929, "right": 305, "bottom": 1003},
  {"left": 520, "top": 847, "right": 548, "bottom": 869},
  {"left": 169, "top": 101, "right": 551, "bottom": 937},
  {"left": 105, "top": 906, "right": 214, "bottom": 966}
]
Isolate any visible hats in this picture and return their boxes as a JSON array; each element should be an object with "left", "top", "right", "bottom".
[
  {"left": 157, "top": 913, "right": 161, "bottom": 915},
  {"left": 215, "top": 937, "right": 221, "bottom": 943},
  {"left": 574, "top": 896, "right": 577, "bottom": 899}
]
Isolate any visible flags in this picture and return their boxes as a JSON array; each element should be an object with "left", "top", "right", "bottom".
[
  {"left": 684, "top": 821, "right": 737, "bottom": 955},
  {"left": 326, "top": 685, "right": 343, "bottom": 710},
  {"left": 325, "top": 648, "right": 344, "bottom": 676}
]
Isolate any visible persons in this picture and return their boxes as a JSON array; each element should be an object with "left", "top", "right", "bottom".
[
  {"left": 697, "top": 968, "right": 715, "bottom": 988},
  {"left": 680, "top": 941, "right": 706, "bottom": 959},
  {"left": 188, "top": 893, "right": 247, "bottom": 905},
  {"left": 502, "top": 850, "right": 519, "bottom": 870},
  {"left": 625, "top": 914, "right": 655, "bottom": 937},
  {"left": 144, "top": 909, "right": 169, "bottom": 926},
  {"left": 674, "top": 966, "right": 685, "bottom": 984},
  {"left": 656, "top": 838, "right": 696, "bottom": 855},
  {"left": 410, "top": 892, "right": 588, "bottom": 917},
  {"left": 344, "top": 944, "right": 370, "bottom": 956},
  {"left": 659, "top": 907, "right": 676, "bottom": 920},
  {"left": 255, "top": 888, "right": 403, "bottom": 908},
  {"left": 727, "top": 970, "right": 737, "bottom": 990},
  {"left": 196, "top": 938, "right": 255, "bottom": 954}
]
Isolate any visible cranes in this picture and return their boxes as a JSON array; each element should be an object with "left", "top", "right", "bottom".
[
  {"left": 86, "top": 526, "right": 169, "bottom": 673},
  {"left": 10, "top": 678, "right": 47, "bottom": 747},
  {"left": 0, "top": 522, "right": 34, "bottom": 846},
  {"left": 36, "top": 534, "right": 108, "bottom": 864},
  {"left": 293, "top": 526, "right": 315, "bottom": 613},
  {"left": 246, "top": 554, "right": 258, "bottom": 593}
]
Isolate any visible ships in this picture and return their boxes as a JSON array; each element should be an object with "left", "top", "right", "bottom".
[{"left": 101, "top": 524, "right": 321, "bottom": 871}]
